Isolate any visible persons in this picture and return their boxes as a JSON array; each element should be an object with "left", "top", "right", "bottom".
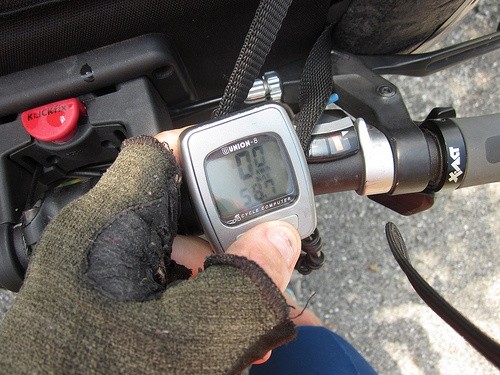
[{"left": 0, "top": 123, "right": 378, "bottom": 375}]
